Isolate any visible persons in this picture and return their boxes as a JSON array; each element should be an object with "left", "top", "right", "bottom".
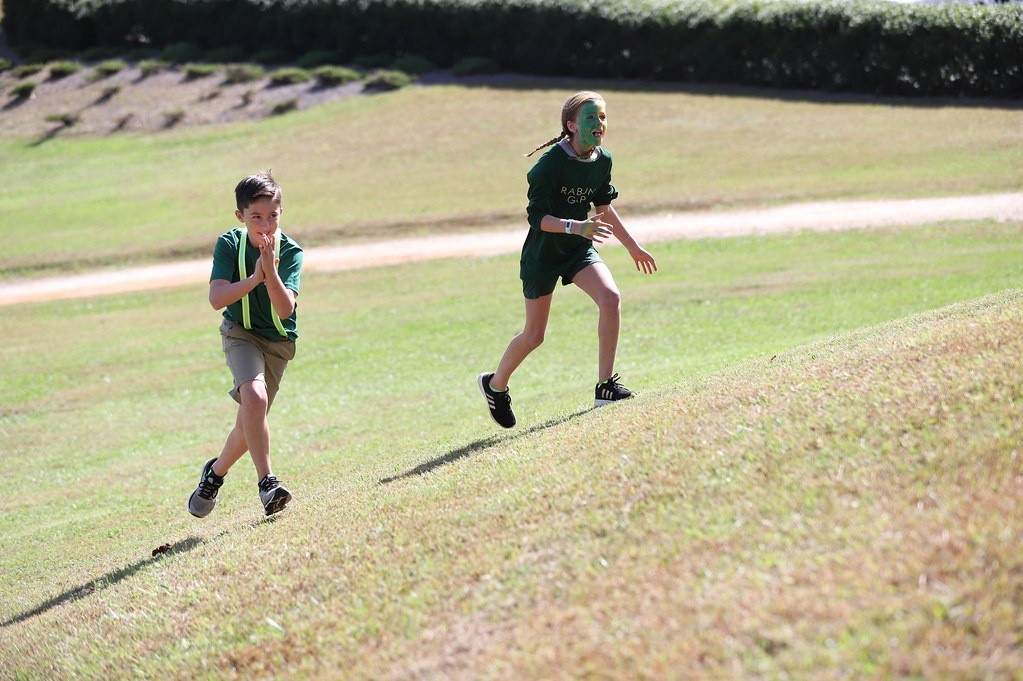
[
  {"left": 188, "top": 168, "right": 304, "bottom": 518},
  {"left": 477, "top": 91, "right": 658, "bottom": 430}
]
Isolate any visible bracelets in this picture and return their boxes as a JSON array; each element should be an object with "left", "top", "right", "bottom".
[{"left": 621, "top": 235, "right": 631, "bottom": 243}]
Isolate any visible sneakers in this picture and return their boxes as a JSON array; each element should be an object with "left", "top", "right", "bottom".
[
  {"left": 594, "top": 373, "right": 636, "bottom": 407},
  {"left": 477, "top": 372, "right": 517, "bottom": 429},
  {"left": 257, "top": 474, "right": 293, "bottom": 516},
  {"left": 187, "top": 457, "right": 229, "bottom": 519}
]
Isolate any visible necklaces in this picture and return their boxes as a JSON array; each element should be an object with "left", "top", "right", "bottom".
[{"left": 567, "top": 142, "right": 593, "bottom": 157}]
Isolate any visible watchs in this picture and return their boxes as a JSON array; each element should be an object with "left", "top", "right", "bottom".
[{"left": 565, "top": 218, "right": 573, "bottom": 235}]
